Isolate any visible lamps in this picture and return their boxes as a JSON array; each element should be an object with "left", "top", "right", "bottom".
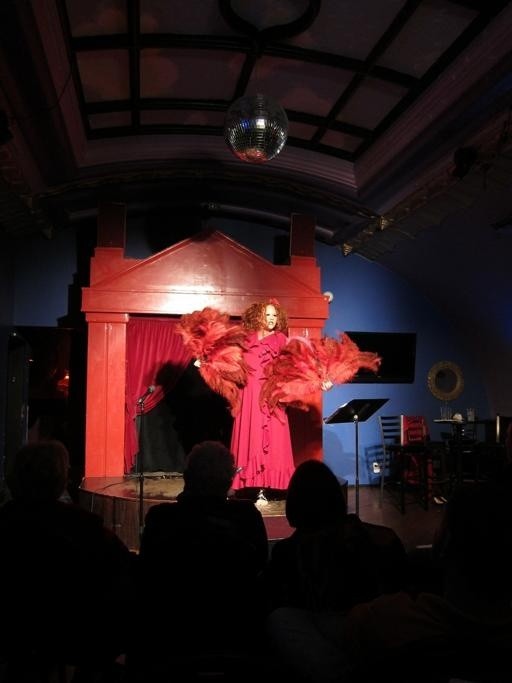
[{"left": 213, "top": 1, "right": 322, "bottom": 164}]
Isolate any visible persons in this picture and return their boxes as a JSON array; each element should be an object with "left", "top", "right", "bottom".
[
  {"left": 0, "top": 438, "right": 131, "bottom": 556},
  {"left": 193, "top": 302, "right": 333, "bottom": 501},
  {"left": 271, "top": 460, "right": 407, "bottom": 562},
  {"left": 139, "top": 440, "right": 269, "bottom": 564}
]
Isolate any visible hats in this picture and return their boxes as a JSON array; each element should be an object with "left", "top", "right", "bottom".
[{"left": 287, "top": 460, "right": 348, "bottom": 528}]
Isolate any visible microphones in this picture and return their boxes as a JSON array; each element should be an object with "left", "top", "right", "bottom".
[{"left": 136, "top": 386, "right": 155, "bottom": 405}]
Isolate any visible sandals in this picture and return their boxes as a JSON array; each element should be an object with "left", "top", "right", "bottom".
[{"left": 256, "top": 489, "right": 269, "bottom": 503}]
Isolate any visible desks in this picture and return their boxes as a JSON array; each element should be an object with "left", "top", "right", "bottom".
[{"left": 388, "top": 442, "right": 443, "bottom": 513}]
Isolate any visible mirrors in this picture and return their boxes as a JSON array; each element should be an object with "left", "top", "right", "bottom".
[{"left": 427, "top": 362, "right": 465, "bottom": 400}]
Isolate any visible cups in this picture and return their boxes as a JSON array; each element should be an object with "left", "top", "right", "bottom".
[{"left": 467, "top": 408, "right": 475, "bottom": 421}]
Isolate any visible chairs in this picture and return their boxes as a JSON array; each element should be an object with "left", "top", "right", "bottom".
[{"left": 378, "top": 415, "right": 402, "bottom": 492}]
